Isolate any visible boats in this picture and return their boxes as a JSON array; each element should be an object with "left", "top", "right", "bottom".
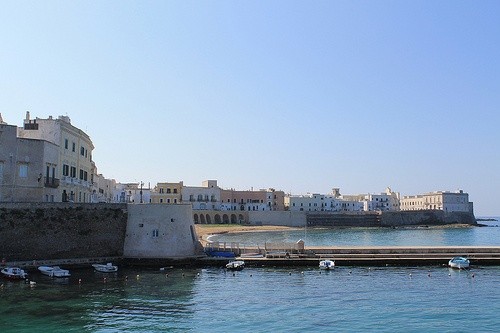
[
  {"left": 92, "top": 263, "right": 118, "bottom": 273},
  {"left": 226, "top": 261, "right": 245, "bottom": 270},
  {"left": 1, "top": 267, "right": 29, "bottom": 280},
  {"left": 448, "top": 258, "right": 470, "bottom": 270},
  {"left": 318, "top": 261, "right": 335, "bottom": 271},
  {"left": 36, "top": 265, "right": 71, "bottom": 277}
]
